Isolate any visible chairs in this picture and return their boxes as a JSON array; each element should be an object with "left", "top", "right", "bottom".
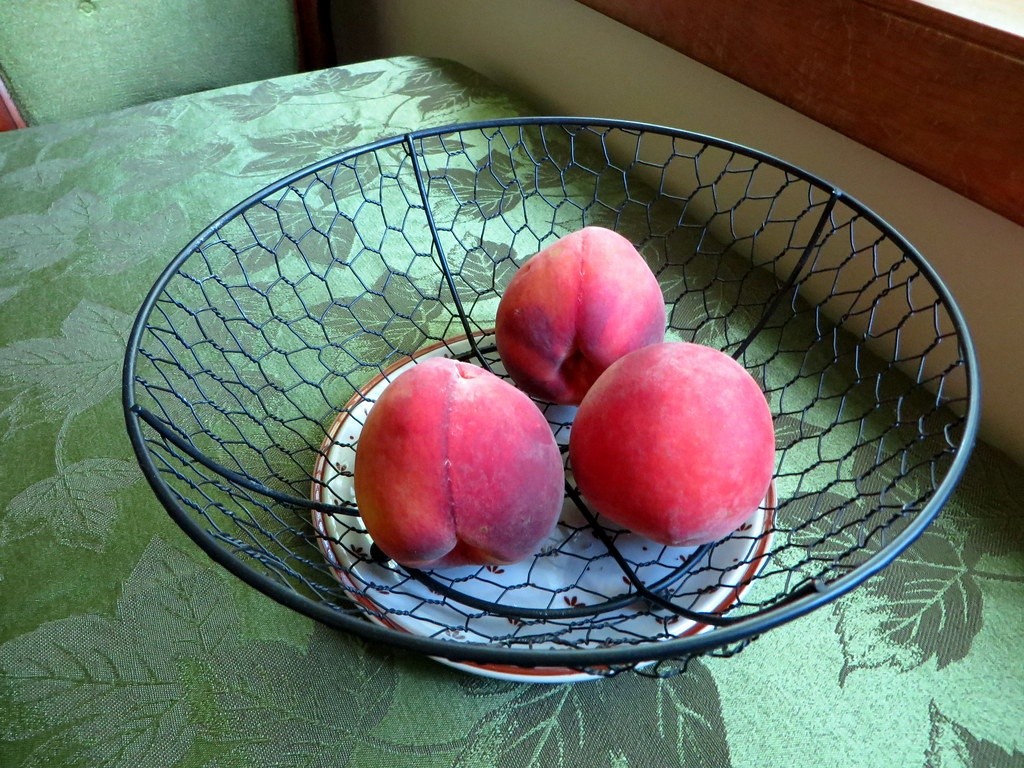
[{"left": 0, "top": 0, "right": 296, "bottom": 134}]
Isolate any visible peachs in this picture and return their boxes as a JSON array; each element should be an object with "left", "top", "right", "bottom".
[{"left": 355, "top": 225, "right": 776, "bottom": 568}]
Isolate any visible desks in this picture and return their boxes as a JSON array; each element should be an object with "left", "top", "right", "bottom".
[{"left": 0, "top": 51, "right": 1024, "bottom": 768}]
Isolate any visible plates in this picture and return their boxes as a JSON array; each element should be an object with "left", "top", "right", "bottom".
[{"left": 309, "top": 329, "right": 778, "bottom": 685}]
